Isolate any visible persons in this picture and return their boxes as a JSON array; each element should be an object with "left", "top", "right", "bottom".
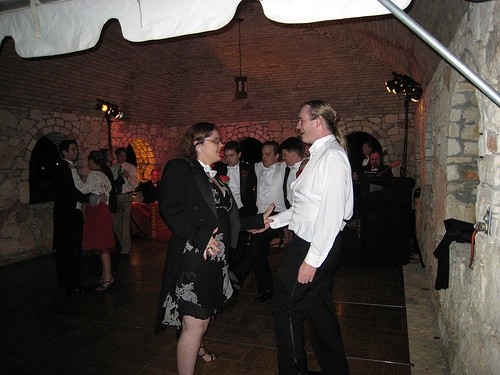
[
  {"left": 246, "top": 99, "right": 355, "bottom": 375},
  {"left": 141, "top": 169, "right": 161, "bottom": 203},
  {"left": 351, "top": 143, "right": 394, "bottom": 241},
  {"left": 99, "top": 147, "right": 136, "bottom": 257},
  {"left": 53, "top": 139, "right": 115, "bottom": 295},
  {"left": 154, "top": 122, "right": 276, "bottom": 375},
  {"left": 214, "top": 137, "right": 309, "bottom": 303}
]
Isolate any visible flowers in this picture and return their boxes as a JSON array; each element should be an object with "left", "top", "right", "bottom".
[
  {"left": 242, "top": 169, "right": 249, "bottom": 178},
  {"left": 219, "top": 175, "right": 230, "bottom": 185}
]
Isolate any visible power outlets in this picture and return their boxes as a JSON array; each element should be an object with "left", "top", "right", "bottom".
[{"left": 484, "top": 211, "right": 492, "bottom": 238}]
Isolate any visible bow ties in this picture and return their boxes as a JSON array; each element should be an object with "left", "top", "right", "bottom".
[
  {"left": 364, "top": 157, "right": 367, "bottom": 159},
  {"left": 204, "top": 164, "right": 217, "bottom": 178}
]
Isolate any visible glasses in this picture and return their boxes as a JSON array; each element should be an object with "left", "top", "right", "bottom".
[{"left": 198, "top": 138, "right": 220, "bottom": 143}]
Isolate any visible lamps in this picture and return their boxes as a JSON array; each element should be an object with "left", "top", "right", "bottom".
[
  {"left": 95, "top": 100, "right": 128, "bottom": 120},
  {"left": 385, "top": 73, "right": 424, "bottom": 102},
  {"left": 235, "top": 19, "right": 247, "bottom": 100}
]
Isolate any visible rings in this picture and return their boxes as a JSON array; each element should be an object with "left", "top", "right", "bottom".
[{"left": 207, "top": 247, "right": 213, "bottom": 250}]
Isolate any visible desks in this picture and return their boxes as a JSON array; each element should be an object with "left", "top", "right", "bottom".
[{"left": 130, "top": 202, "right": 172, "bottom": 241}]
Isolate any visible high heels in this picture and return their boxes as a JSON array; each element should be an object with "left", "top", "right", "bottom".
[{"left": 198, "top": 346, "right": 216, "bottom": 362}]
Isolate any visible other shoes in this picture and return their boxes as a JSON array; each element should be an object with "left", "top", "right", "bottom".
[
  {"left": 64, "top": 285, "right": 93, "bottom": 296},
  {"left": 95, "top": 277, "right": 117, "bottom": 292},
  {"left": 255, "top": 293, "right": 274, "bottom": 303}
]
